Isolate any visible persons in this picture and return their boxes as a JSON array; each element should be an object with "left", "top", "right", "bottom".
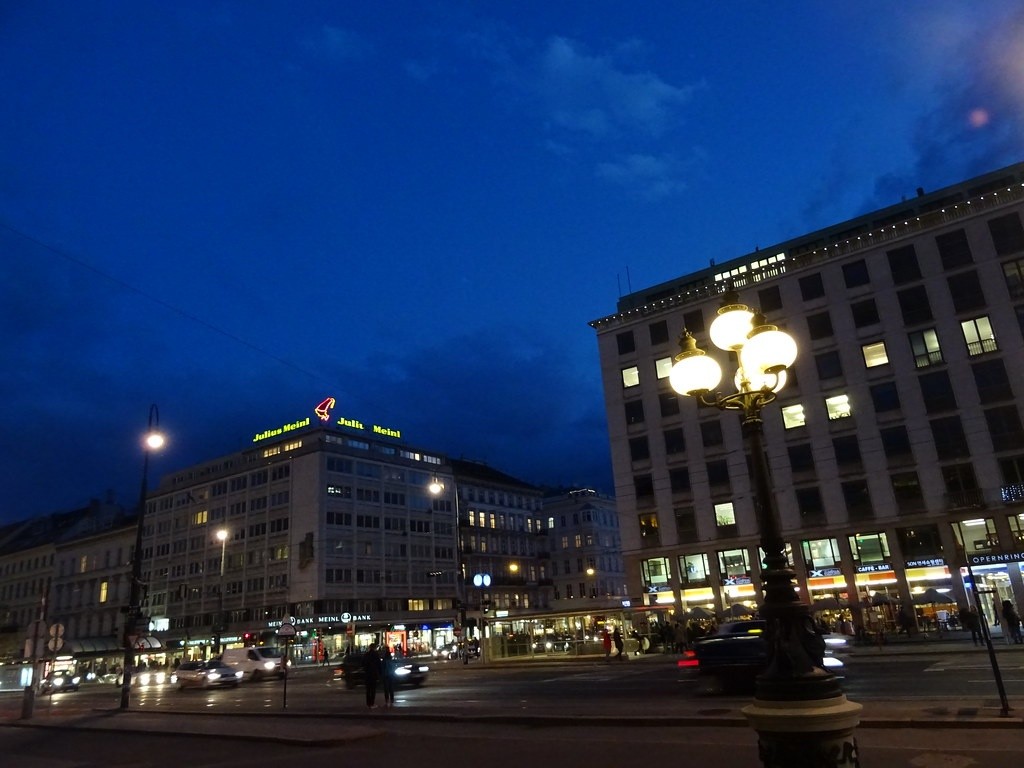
[
  {"left": 612, "top": 626, "right": 624, "bottom": 656},
  {"left": 959, "top": 607, "right": 985, "bottom": 646},
  {"left": 463, "top": 636, "right": 470, "bottom": 648},
  {"left": 1001, "top": 600, "right": 1023, "bottom": 645},
  {"left": 382, "top": 651, "right": 395, "bottom": 704},
  {"left": 472, "top": 636, "right": 480, "bottom": 658},
  {"left": 816, "top": 615, "right": 831, "bottom": 634},
  {"left": 323, "top": 647, "right": 329, "bottom": 666},
  {"left": 601, "top": 628, "right": 612, "bottom": 656},
  {"left": 646, "top": 620, "right": 719, "bottom": 651},
  {"left": 362, "top": 643, "right": 384, "bottom": 710},
  {"left": 512, "top": 626, "right": 597, "bottom": 642},
  {"left": 992, "top": 597, "right": 1000, "bottom": 626},
  {"left": 839, "top": 613, "right": 846, "bottom": 634}
]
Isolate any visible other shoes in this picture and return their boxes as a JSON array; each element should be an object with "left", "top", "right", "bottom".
[{"left": 366, "top": 704, "right": 378, "bottom": 709}]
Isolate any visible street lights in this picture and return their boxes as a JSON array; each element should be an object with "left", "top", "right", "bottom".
[
  {"left": 428, "top": 463, "right": 466, "bottom": 663},
  {"left": 472, "top": 571, "right": 492, "bottom": 662},
  {"left": 118, "top": 401, "right": 165, "bottom": 708},
  {"left": 214, "top": 529, "right": 229, "bottom": 653},
  {"left": 670, "top": 291, "right": 864, "bottom": 768}
]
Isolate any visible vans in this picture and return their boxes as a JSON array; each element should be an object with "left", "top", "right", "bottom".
[{"left": 220, "top": 644, "right": 292, "bottom": 682}]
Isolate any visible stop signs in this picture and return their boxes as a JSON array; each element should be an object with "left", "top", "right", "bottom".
[{"left": 453, "top": 628, "right": 461, "bottom": 636}]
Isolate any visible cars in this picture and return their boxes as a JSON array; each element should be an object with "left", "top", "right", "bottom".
[
  {"left": 676, "top": 621, "right": 851, "bottom": 695},
  {"left": 169, "top": 659, "right": 244, "bottom": 690},
  {"left": 339, "top": 651, "right": 431, "bottom": 688},
  {"left": 39, "top": 669, "right": 79, "bottom": 696}
]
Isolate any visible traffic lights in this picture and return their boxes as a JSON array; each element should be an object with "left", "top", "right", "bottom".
[{"left": 244, "top": 633, "right": 251, "bottom": 639}]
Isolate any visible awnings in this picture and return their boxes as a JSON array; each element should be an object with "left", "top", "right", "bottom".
[{"left": 70, "top": 636, "right": 162, "bottom": 656}]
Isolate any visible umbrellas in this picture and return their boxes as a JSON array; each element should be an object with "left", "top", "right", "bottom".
[
  {"left": 670, "top": 598, "right": 872, "bottom": 625},
  {"left": 873, "top": 592, "right": 900, "bottom": 619},
  {"left": 911, "top": 588, "right": 953, "bottom": 612}
]
